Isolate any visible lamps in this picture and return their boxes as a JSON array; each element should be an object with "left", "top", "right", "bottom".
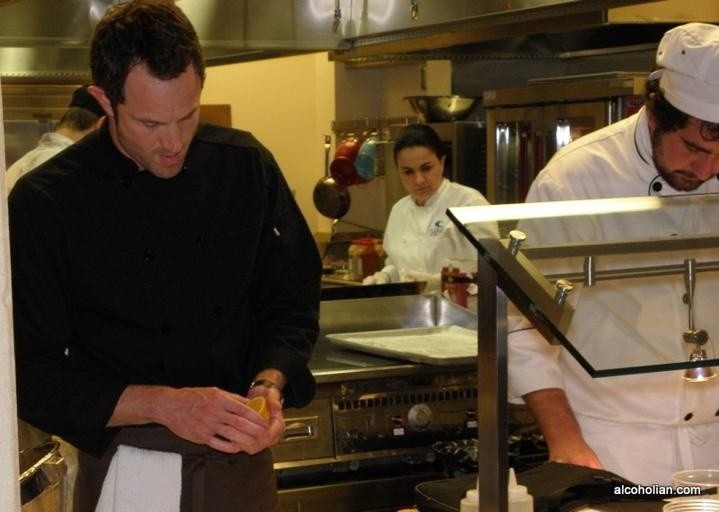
[{"left": 682, "top": 258, "right": 718, "bottom": 383}]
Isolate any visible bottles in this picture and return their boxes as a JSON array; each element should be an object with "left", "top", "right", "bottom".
[{"left": 348, "top": 237, "right": 385, "bottom": 283}]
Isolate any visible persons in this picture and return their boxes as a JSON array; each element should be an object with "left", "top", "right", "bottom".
[
  {"left": 468, "top": 16, "right": 717, "bottom": 492},
  {"left": 350, "top": 114, "right": 507, "bottom": 308},
  {"left": 8, "top": 2, "right": 321, "bottom": 509},
  {"left": 4, "top": 86, "right": 115, "bottom": 206}
]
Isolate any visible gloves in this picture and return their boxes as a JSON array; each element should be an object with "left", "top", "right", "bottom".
[
  {"left": 362, "top": 271, "right": 391, "bottom": 286},
  {"left": 402, "top": 271, "right": 443, "bottom": 295}
]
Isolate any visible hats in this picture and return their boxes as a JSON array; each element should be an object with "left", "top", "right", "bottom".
[
  {"left": 68, "top": 84, "right": 106, "bottom": 117},
  {"left": 655, "top": 21, "right": 718, "bottom": 123}
]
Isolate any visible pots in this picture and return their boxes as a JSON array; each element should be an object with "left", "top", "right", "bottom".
[{"left": 311, "top": 129, "right": 387, "bottom": 220}]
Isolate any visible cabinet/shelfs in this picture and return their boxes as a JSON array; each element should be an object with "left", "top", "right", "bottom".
[{"left": 486, "top": 99, "right": 616, "bottom": 205}]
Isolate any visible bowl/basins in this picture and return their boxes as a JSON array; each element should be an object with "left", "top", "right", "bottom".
[{"left": 401, "top": 93, "right": 483, "bottom": 123}]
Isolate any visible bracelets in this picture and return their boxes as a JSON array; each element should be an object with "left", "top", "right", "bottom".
[{"left": 243, "top": 374, "right": 286, "bottom": 402}]
